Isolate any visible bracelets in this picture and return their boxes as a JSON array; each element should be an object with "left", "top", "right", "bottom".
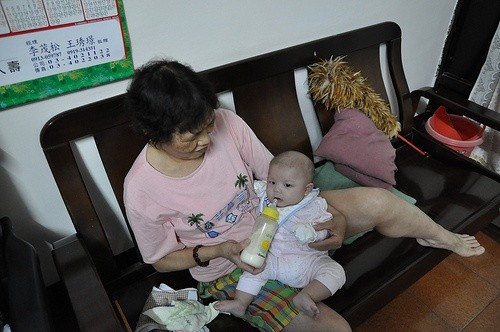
[{"left": 193, "top": 245, "right": 210, "bottom": 269}]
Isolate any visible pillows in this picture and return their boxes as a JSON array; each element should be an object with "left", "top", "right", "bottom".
[
  {"left": 312, "top": 108, "right": 398, "bottom": 185},
  {"left": 334, "top": 163, "right": 392, "bottom": 192}
]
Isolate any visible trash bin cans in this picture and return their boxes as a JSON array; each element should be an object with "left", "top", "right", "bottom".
[{"left": 425, "top": 105, "right": 486, "bottom": 158}]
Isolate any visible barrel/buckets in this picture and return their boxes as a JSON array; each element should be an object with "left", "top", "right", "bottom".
[{"left": 425, "top": 114, "right": 486, "bottom": 156}]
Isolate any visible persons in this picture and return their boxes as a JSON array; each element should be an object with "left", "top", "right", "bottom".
[
  {"left": 212, "top": 151, "right": 347, "bottom": 319},
  {"left": 122, "top": 57, "right": 487, "bottom": 332}
]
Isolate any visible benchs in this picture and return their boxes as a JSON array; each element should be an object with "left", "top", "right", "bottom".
[{"left": 39, "top": 21, "right": 500, "bottom": 332}]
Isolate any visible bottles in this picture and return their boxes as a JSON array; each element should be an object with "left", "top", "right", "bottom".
[{"left": 239, "top": 198, "right": 280, "bottom": 268}]
